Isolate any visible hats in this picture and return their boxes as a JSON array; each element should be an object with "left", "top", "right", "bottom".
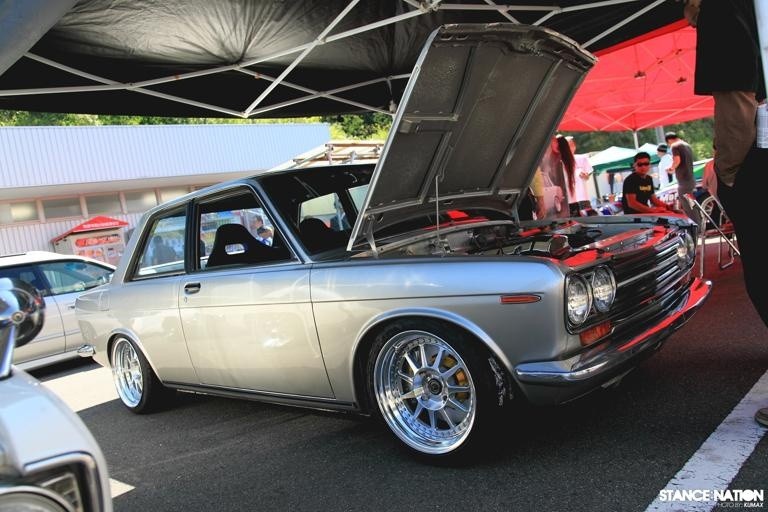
[{"left": 657, "top": 143, "right": 667, "bottom": 152}]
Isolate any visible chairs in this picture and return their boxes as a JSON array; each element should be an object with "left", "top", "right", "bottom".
[{"left": 687, "top": 186, "right": 741, "bottom": 279}]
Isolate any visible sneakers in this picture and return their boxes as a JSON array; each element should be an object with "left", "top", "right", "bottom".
[{"left": 755, "top": 408, "right": 768, "bottom": 428}]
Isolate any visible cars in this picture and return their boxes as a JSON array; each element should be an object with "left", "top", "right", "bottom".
[
  {"left": 0, "top": 248, "right": 118, "bottom": 376},
  {"left": 1, "top": 272, "right": 118, "bottom": 512},
  {"left": 646, "top": 175, "right": 721, "bottom": 233},
  {"left": 65, "top": 18, "right": 720, "bottom": 474}
]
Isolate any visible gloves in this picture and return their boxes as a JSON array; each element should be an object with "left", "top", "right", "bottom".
[{"left": 668, "top": 173, "right": 672, "bottom": 182}]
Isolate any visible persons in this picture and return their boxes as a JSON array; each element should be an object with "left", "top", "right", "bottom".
[
  {"left": 330, "top": 199, "right": 352, "bottom": 231},
  {"left": 153, "top": 236, "right": 177, "bottom": 265},
  {"left": 518, "top": 132, "right": 718, "bottom": 237},
  {"left": 250, "top": 216, "right": 274, "bottom": 247},
  {"left": 677, "top": 0, "right": 768, "bottom": 428}
]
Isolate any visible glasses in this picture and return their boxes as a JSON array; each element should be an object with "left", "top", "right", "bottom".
[{"left": 637, "top": 162, "right": 649, "bottom": 167}]
